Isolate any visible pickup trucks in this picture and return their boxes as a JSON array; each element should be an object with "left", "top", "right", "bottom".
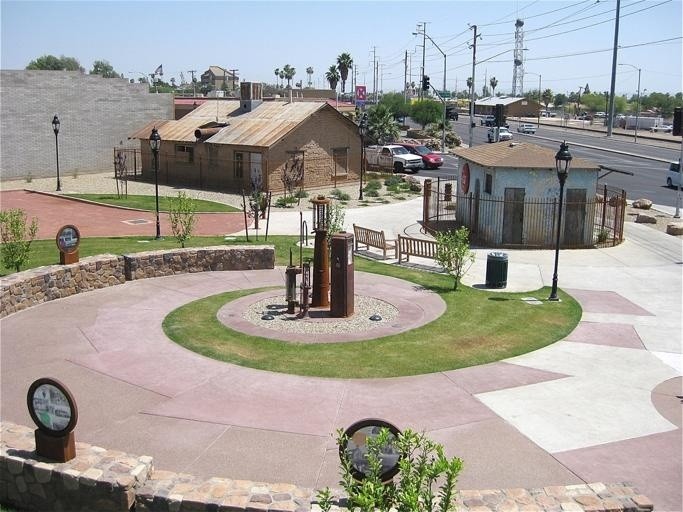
[{"left": 517, "top": 125, "right": 536, "bottom": 134}]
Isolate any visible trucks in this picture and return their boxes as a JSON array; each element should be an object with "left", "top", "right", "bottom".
[
  {"left": 446, "top": 106, "right": 457, "bottom": 121},
  {"left": 362, "top": 145, "right": 425, "bottom": 174}
]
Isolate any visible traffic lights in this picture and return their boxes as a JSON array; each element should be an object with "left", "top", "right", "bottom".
[{"left": 421, "top": 75, "right": 430, "bottom": 92}]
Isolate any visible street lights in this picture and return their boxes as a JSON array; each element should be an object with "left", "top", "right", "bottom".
[
  {"left": 547, "top": 141, "right": 572, "bottom": 301},
  {"left": 149, "top": 125, "right": 164, "bottom": 239},
  {"left": 618, "top": 63, "right": 642, "bottom": 144},
  {"left": 356, "top": 121, "right": 366, "bottom": 200},
  {"left": 412, "top": 32, "right": 447, "bottom": 105},
  {"left": 52, "top": 115, "right": 62, "bottom": 192},
  {"left": 471, "top": 48, "right": 529, "bottom": 122},
  {"left": 525, "top": 72, "right": 541, "bottom": 130}
]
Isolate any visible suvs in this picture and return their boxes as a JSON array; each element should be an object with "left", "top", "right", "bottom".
[{"left": 481, "top": 115, "right": 496, "bottom": 126}]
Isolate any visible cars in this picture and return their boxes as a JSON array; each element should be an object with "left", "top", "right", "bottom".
[
  {"left": 491, "top": 118, "right": 509, "bottom": 129},
  {"left": 574, "top": 111, "right": 607, "bottom": 119},
  {"left": 539, "top": 110, "right": 557, "bottom": 117},
  {"left": 666, "top": 160, "right": 683, "bottom": 190},
  {"left": 616, "top": 114, "right": 626, "bottom": 120},
  {"left": 650, "top": 125, "right": 672, "bottom": 133},
  {"left": 488, "top": 127, "right": 512, "bottom": 142},
  {"left": 337, "top": 92, "right": 379, "bottom": 105}
]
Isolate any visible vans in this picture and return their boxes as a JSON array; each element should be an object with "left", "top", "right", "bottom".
[{"left": 393, "top": 142, "right": 444, "bottom": 169}]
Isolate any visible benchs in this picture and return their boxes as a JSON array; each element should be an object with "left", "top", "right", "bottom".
[
  {"left": 398, "top": 234, "right": 460, "bottom": 271},
  {"left": 353, "top": 224, "right": 399, "bottom": 260}
]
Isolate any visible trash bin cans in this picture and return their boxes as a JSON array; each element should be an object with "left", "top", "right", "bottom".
[{"left": 485, "top": 252, "right": 508, "bottom": 288}]
